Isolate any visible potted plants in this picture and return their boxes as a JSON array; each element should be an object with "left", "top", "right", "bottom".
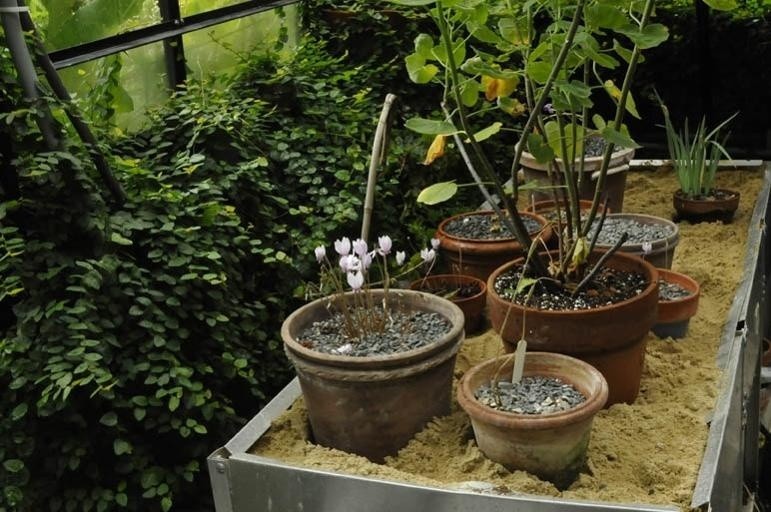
[
  {"left": 405, "top": 0, "right": 663, "bottom": 411},
  {"left": 646, "top": 85, "right": 742, "bottom": 225}
]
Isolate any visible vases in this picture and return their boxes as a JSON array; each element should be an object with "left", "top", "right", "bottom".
[
  {"left": 526, "top": 195, "right": 611, "bottom": 227},
  {"left": 562, "top": 212, "right": 682, "bottom": 273},
  {"left": 511, "top": 139, "right": 637, "bottom": 213},
  {"left": 433, "top": 210, "right": 553, "bottom": 284},
  {"left": 648, "top": 263, "right": 699, "bottom": 339},
  {"left": 416, "top": 272, "right": 489, "bottom": 334},
  {"left": 464, "top": 351, "right": 612, "bottom": 489},
  {"left": 280, "top": 288, "right": 466, "bottom": 466}
]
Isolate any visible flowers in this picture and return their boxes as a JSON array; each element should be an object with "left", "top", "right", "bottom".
[{"left": 303, "top": 235, "right": 440, "bottom": 342}]
